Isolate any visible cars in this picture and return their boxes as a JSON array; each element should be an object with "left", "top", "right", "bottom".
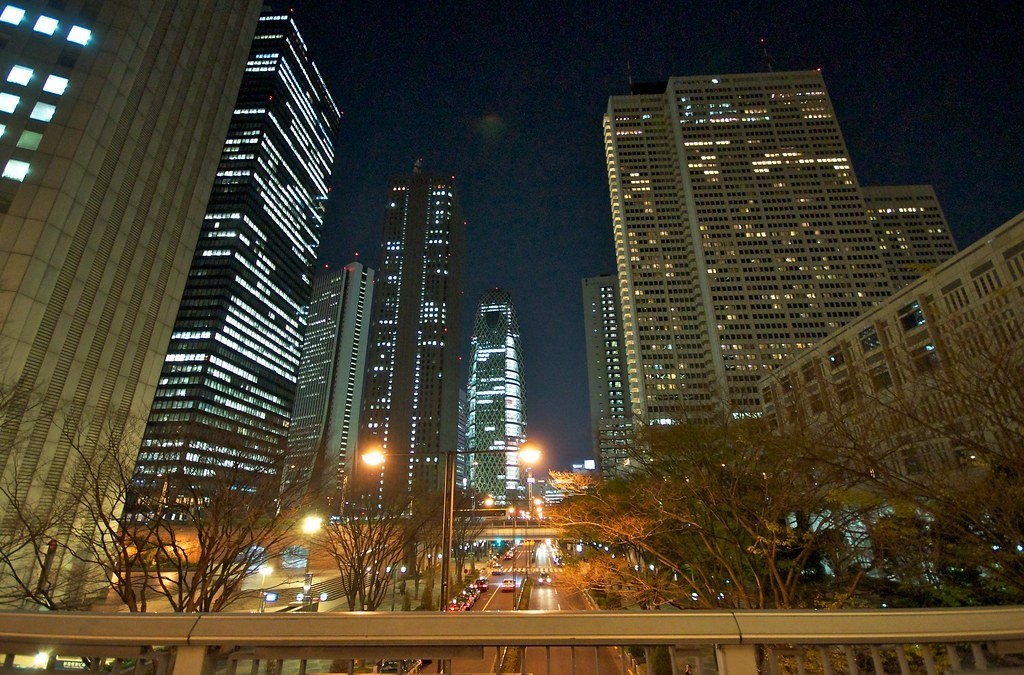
[
  {"left": 501, "top": 578, "right": 516, "bottom": 593},
  {"left": 491, "top": 564, "right": 504, "bottom": 575},
  {"left": 504, "top": 545, "right": 517, "bottom": 560},
  {"left": 381, "top": 658, "right": 418, "bottom": 675},
  {"left": 445, "top": 576, "right": 490, "bottom": 611}
]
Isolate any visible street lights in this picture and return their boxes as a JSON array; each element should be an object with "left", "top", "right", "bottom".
[
  {"left": 446, "top": 446, "right": 540, "bottom": 611},
  {"left": 363, "top": 447, "right": 449, "bottom": 612}
]
